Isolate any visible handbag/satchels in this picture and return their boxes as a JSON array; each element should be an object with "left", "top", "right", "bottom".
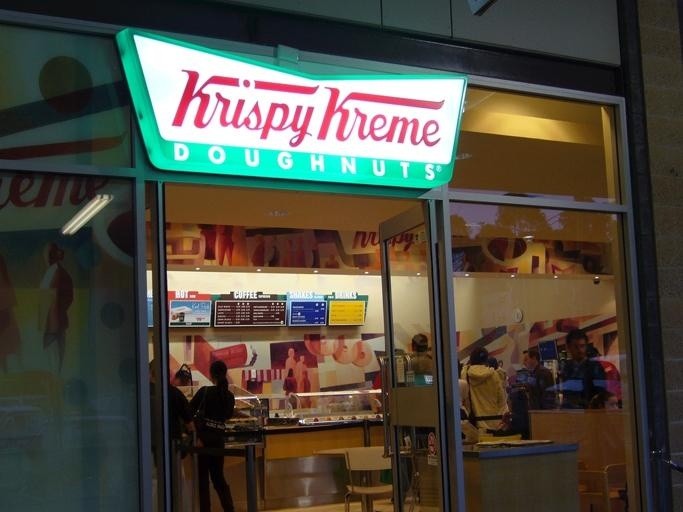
[
  {"left": 193, "top": 385, "right": 209, "bottom": 442},
  {"left": 465, "top": 365, "right": 477, "bottom": 427}
]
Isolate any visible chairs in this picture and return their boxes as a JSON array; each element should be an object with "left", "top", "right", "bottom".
[
  {"left": 577, "top": 470, "right": 609, "bottom": 511},
  {"left": 345, "top": 447, "right": 393, "bottom": 512},
  {"left": 605, "top": 462, "right": 626, "bottom": 495}
]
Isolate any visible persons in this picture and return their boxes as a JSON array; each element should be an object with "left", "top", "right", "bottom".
[
  {"left": 286, "top": 348, "right": 296, "bottom": 369},
  {"left": 299, "top": 369, "right": 311, "bottom": 393},
  {"left": 148, "top": 358, "right": 191, "bottom": 512},
  {"left": 460, "top": 344, "right": 510, "bottom": 437},
  {"left": 402, "top": 334, "right": 434, "bottom": 385},
  {"left": 189, "top": 361, "right": 236, "bottom": 512},
  {"left": 297, "top": 355, "right": 307, "bottom": 373},
  {"left": 283, "top": 368, "right": 298, "bottom": 394},
  {"left": 589, "top": 390, "right": 618, "bottom": 412},
  {"left": 560, "top": 328, "right": 607, "bottom": 410},
  {"left": 523, "top": 350, "right": 556, "bottom": 411}
]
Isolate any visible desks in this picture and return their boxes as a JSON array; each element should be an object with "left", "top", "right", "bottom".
[
  {"left": 313, "top": 446, "right": 346, "bottom": 458},
  {"left": 410, "top": 434, "right": 581, "bottom": 512}
]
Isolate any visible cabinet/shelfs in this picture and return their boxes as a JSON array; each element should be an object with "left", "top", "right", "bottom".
[
  {"left": 296, "top": 388, "right": 385, "bottom": 425},
  {"left": 221, "top": 384, "right": 262, "bottom": 444}
]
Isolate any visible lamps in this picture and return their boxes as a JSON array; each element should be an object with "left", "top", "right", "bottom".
[{"left": 60, "top": 193, "right": 113, "bottom": 236}]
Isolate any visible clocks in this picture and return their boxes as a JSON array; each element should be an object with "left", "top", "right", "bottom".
[{"left": 511, "top": 308, "right": 523, "bottom": 323}]
